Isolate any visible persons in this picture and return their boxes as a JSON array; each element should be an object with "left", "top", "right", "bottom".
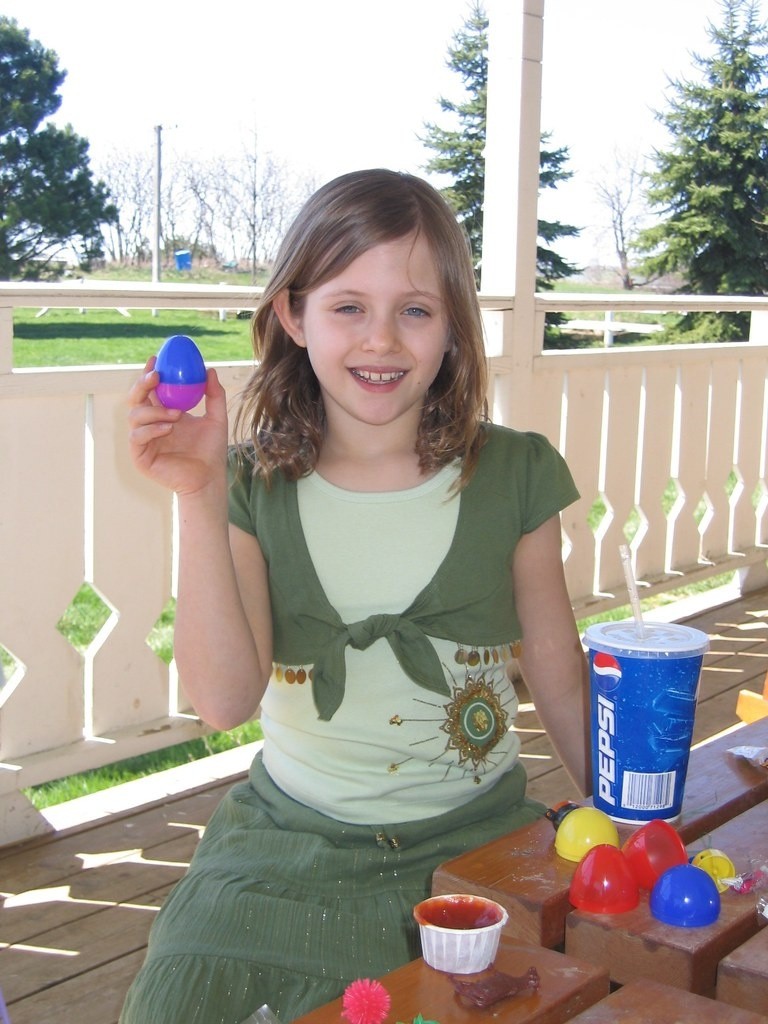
[{"left": 116, "top": 168, "right": 592, "bottom": 1024}]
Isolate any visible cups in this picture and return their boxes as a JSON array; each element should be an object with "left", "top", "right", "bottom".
[
  {"left": 583, "top": 620, "right": 708, "bottom": 825},
  {"left": 414, "top": 895, "right": 507, "bottom": 976}
]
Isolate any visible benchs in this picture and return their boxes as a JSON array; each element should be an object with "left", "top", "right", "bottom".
[{"left": 293, "top": 716, "right": 768, "bottom": 1024}]
